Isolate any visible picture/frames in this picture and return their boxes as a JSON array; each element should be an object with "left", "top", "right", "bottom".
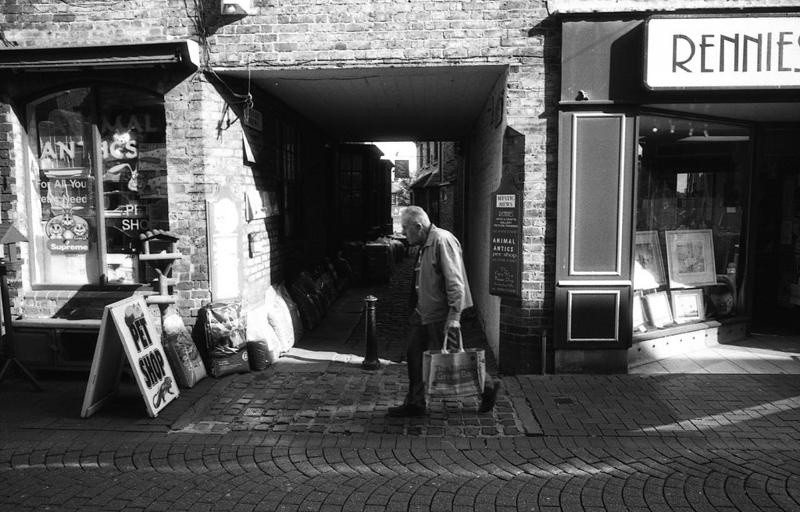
[
  {"left": 646, "top": 290, "right": 673, "bottom": 328},
  {"left": 632, "top": 230, "right": 667, "bottom": 288},
  {"left": 664, "top": 229, "right": 717, "bottom": 289},
  {"left": 669, "top": 289, "right": 705, "bottom": 323}
]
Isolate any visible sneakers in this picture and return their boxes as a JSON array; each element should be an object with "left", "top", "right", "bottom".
[
  {"left": 480, "top": 379, "right": 500, "bottom": 412},
  {"left": 387, "top": 405, "right": 426, "bottom": 419}
]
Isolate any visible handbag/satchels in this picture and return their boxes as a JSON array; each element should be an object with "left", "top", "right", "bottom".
[{"left": 422, "top": 329, "right": 485, "bottom": 413}]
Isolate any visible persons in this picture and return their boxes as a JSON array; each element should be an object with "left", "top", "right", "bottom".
[{"left": 388, "top": 206, "right": 502, "bottom": 417}]
